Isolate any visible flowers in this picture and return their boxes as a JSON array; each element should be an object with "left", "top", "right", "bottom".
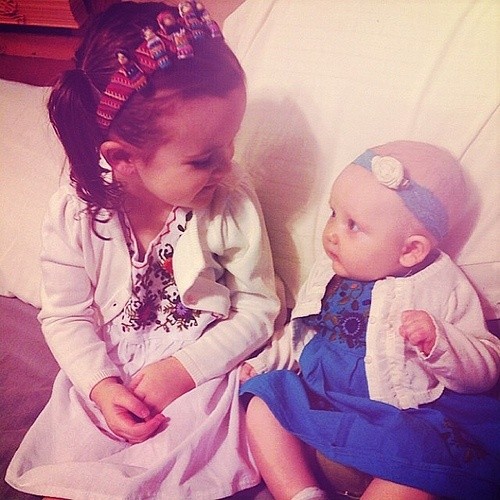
[{"left": 370, "top": 155, "right": 404, "bottom": 189}]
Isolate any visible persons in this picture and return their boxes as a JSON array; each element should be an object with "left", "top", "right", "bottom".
[
  {"left": 4, "top": 0, "right": 282, "bottom": 500},
  {"left": 238, "top": 140, "right": 500, "bottom": 500}
]
